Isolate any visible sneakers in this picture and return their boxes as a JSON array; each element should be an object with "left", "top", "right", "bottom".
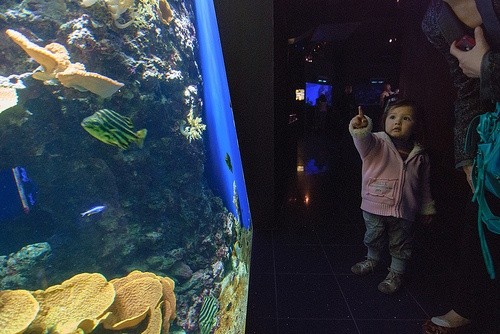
[
  {"left": 378, "top": 270, "right": 407, "bottom": 295},
  {"left": 351, "top": 256, "right": 388, "bottom": 276}
]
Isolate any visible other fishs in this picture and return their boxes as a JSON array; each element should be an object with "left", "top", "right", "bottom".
[
  {"left": 81, "top": 108, "right": 148, "bottom": 150},
  {"left": 80, "top": 206, "right": 107, "bottom": 216}
]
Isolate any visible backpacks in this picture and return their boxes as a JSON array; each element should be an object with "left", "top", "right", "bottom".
[{"left": 464, "top": 102, "right": 500, "bottom": 279}]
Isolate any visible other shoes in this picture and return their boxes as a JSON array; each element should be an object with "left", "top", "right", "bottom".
[{"left": 421, "top": 309, "right": 476, "bottom": 334}]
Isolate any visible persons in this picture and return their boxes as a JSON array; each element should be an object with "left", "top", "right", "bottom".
[
  {"left": 349, "top": 98, "right": 438, "bottom": 297},
  {"left": 420, "top": 0, "right": 500, "bottom": 329},
  {"left": 317, "top": 82, "right": 400, "bottom": 135}
]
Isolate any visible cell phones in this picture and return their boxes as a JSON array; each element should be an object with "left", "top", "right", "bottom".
[{"left": 455, "top": 33, "right": 476, "bottom": 52}]
